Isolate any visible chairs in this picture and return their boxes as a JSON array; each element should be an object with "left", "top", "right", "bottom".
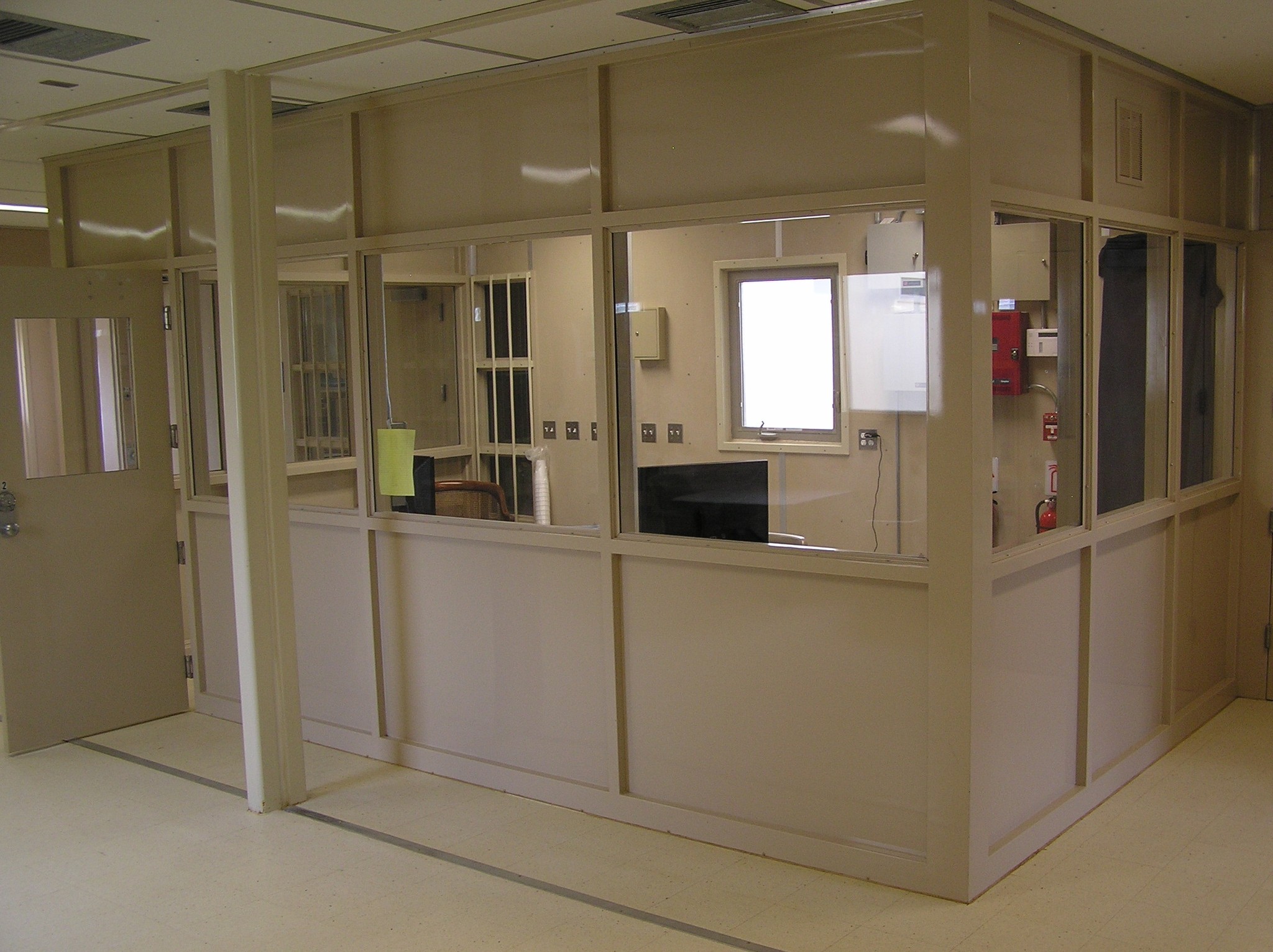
[{"left": 435, "top": 480, "right": 516, "bottom": 523}]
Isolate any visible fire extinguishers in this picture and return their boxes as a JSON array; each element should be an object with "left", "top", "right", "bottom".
[{"left": 1035, "top": 494, "right": 1058, "bottom": 533}]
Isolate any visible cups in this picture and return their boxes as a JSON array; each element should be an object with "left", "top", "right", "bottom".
[{"left": 534, "top": 460, "right": 550, "bottom": 524}]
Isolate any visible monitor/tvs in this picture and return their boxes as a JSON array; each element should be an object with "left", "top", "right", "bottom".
[
  {"left": 638, "top": 459, "right": 768, "bottom": 542},
  {"left": 390, "top": 454, "right": 435, "bottom": 515}
]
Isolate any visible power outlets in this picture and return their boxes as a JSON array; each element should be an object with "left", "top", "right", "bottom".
[{"left": 859, "top": 429, "right": 877, "bottom": 450}]
